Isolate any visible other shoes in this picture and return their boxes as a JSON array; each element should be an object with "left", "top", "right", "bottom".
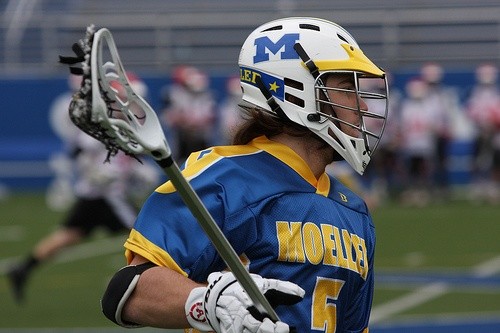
[{"left": 5, "top": 260, "right": 34, "bottom": 302}]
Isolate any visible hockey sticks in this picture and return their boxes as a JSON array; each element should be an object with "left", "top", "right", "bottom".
[{"left": 57, "top": 22, "right": 281, "bottom": 322}]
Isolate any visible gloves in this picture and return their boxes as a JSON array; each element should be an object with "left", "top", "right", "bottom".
[{"left": 184, "top": 270, "right": 305, "bottom": 332}]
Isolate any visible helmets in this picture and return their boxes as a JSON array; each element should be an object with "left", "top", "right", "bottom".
[{"left": 238, "top": 16, "right": 390, "bottom": 175}]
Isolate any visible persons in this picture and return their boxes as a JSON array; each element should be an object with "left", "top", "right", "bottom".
[
  {"left": 5, "top": 69, "right": 148, "bottom": 306},
  {"left": 161, "top": 61, "right": 500, "bottom": 210},
  {"left": 106, "top": 16, "right": 390, "bottom": 333}
]
